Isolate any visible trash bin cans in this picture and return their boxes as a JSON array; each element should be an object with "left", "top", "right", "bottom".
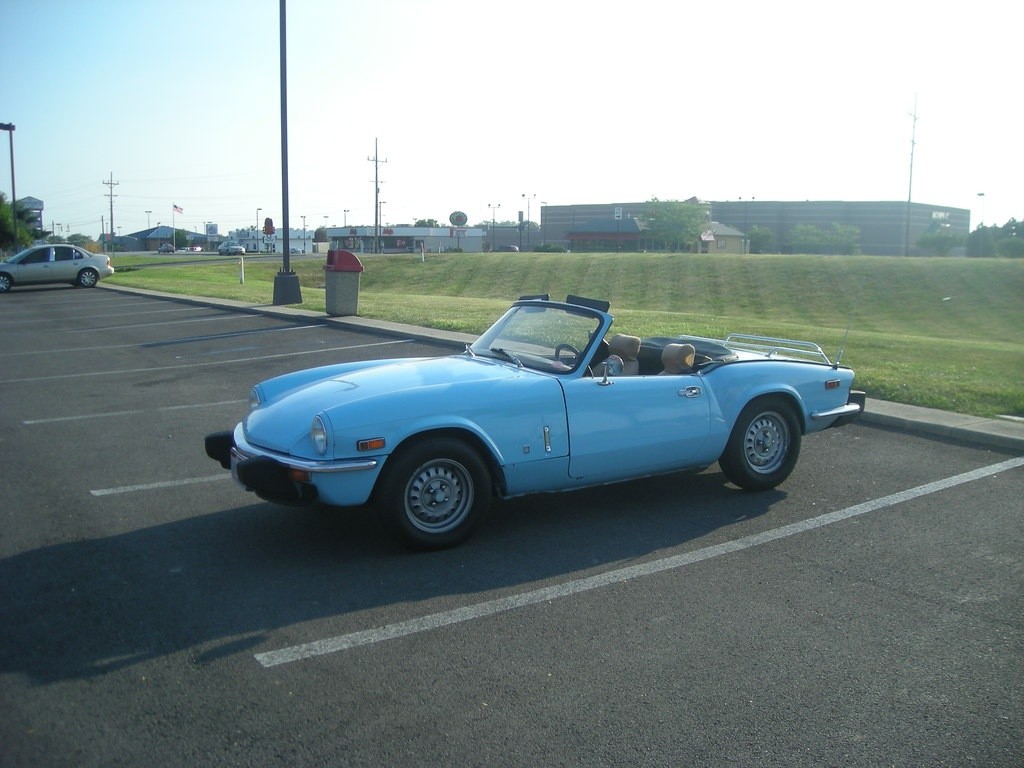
[{"left": 324, "top": 250, "right": 364, "bottom": 317}]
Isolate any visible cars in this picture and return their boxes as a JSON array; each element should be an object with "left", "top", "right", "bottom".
[
  {"left": 289, "top": 247, "right": 302, "bottom": 254},
  {"left": 0, "top": 243, "right": 114, "bottom": 293},
  {"left": 157, "top": 243, "right": 174, "bottom": 254},
  {"left": 203, "top": 293, "right": 868, "bottom": 552},
  {"left": 494, "top": 245, "right": 519, "bottom": 252},
  {"left": 218, "top": 241, "right": 245, "bottom": 256}
]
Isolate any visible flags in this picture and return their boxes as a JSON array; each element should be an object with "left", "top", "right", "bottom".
[{"left": 174, "top": 205, "right": 184, "bottom": 215}]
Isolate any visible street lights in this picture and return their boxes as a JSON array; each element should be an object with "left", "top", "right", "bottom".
[
  {"left": 488, "top": 203, "right": 500, "bottom": 253},
  {"left": 145, "top": 210, "right": 152, "bottom": 229},
  {"left": 117, "top": 227, "right": 122, "bottom": 251},
  {"left": 256, "top": 208, "right": 262, "bottom": 253},
  {"left": 0, "top": 122, "right": 18, "bottom": 256},
  {"left": 522, "top": 193, "right": 536, "bottom": 252},
  {"left": 301, "top": 216, "right": 306, "bottom": 254},
  {"left": 157, "top": 222, "right": 161, "bottom": 254},
  {"left": 977, "top": 193, "right": 985, "bottom": 256},
  {"left": 379, "top": 201, "right": 386, "bottom": 254},
  {"left": 52, "top": 220, "right": 61, "bottom": 244},
  {"left": 344, "top": 210, "right": 349, "bottom": 227}
]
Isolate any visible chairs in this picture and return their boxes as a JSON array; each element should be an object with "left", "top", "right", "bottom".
[
  {"left": 587, "top": 334, "right": 642, "bottom": 375},
  {"left": 657, "top": 343, "right": 695, "bottom": 376}
]
